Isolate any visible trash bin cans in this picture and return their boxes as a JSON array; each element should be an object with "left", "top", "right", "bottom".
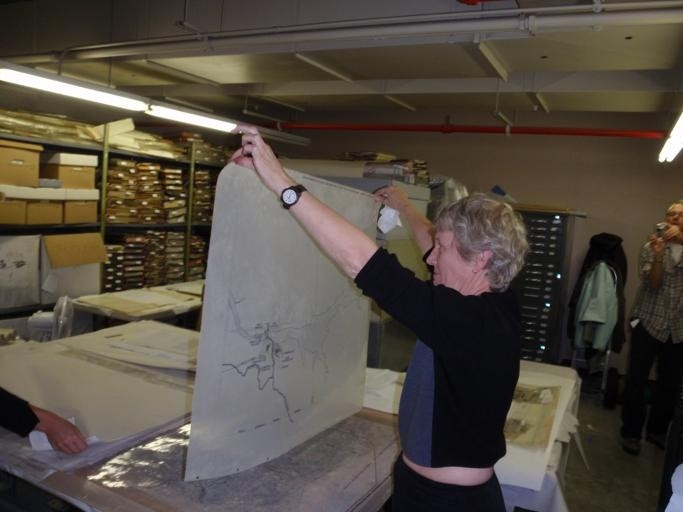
[{"left": 279, "top": 184, "right": 306, "bottom": 209}]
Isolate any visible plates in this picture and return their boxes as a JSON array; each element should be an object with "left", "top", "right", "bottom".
[{"left": 515, "top": 210, "right": 574, "bottom": 363}]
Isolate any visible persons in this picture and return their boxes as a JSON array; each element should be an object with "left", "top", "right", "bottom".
[
  {"left": 230, "top": 124, "right": 531, "bottom": 512},
  {"left": 0, "top": 387, "right": 88, "bottom": 454},
  {"left": 618, "top": 199, "right": 683, "bottom": 456}
]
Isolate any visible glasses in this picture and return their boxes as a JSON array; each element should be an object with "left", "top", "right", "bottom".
[
  {"left": 144, "top": 104, "right": 237, "bottom": 133},
  {"left": 658, "top": 111, "right": 683, "bottom": 162},
  {"left": 0, "top": 61, "right": 149, "bottom": 113},
  {"left": 237, "top": 120, "right": 311, "bottom": 147}
]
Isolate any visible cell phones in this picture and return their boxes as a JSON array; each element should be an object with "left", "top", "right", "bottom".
[{"left": 654, "top": 223, "right": 667, "bottom": 238}]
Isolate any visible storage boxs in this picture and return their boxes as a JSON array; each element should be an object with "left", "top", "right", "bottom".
[{"left": 0, "top": 140, "right": 106, "bottom": 344}]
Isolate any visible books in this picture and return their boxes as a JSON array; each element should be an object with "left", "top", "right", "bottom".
[{"left": 0, "top": 140, "right": 106, "bottom": 344}]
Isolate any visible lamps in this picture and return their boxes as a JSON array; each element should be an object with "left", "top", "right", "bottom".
[
  {"left": 0, "top": 61, "right": 149, "bottom": 113},
  {"left": 144, "top": 104, "right": 237, "bottom": 133},
  {"left": 658, "top": 111, "right": 683, "bottom": 162},
  {"left": 237, "top": 120, "right": 311, "bottom": 147}
]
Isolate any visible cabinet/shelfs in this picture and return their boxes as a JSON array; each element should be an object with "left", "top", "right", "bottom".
[{"left": 515, "top": 210, "right": 574, "bottom": 363}]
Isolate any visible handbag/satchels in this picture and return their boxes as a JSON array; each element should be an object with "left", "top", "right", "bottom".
[{"left": 655, "top": 259, "right": 663, "bottom": 265}]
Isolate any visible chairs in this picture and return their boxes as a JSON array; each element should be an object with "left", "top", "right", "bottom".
[{"left": 621, "top": 437, "right": 640, "bottom": 454}]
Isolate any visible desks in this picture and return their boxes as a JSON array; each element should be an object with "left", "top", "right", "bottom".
[{"left": 1, "top": 280, "right": 589, "bottom": 512}]
